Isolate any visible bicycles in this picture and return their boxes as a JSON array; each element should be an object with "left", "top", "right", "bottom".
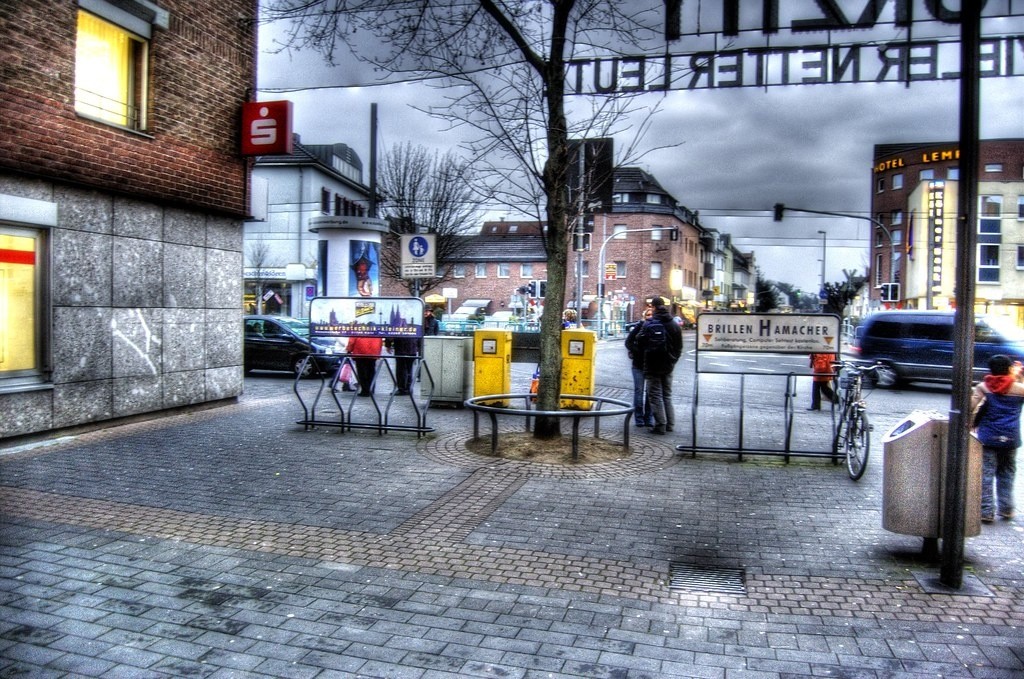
[{"left": 828, "top": 360, "right": 891, "bottom": 481}]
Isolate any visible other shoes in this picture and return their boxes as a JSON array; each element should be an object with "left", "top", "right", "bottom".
[
  {"left": 357, "top": 392, "right": 370, "bottom": 397},
  {"left": 980, "top": 512, "right": 994, "bottom": 522},
  {"left": 648, "top": 429, "right": 665, "bottom": 435},
  {"left": 666, "top": 429, "right": 673, "bottom": 432},
  {"left": 644, "top": 424, "right": 654, "bottom": 427},
  {"left": 342, "top": 386, "right": 356, "bottom": 391},
  {"left": 996, "top": 510, "right": 1016, "bottom": 518},
  {"left": 390, "top": 389, "right": 409, "bottom": 396},
  {"left": 328, "top": 384, "right": 339, "bottom": 393},
  {"left": 637, "top": 424, "right": 644, "bottom": 428}
]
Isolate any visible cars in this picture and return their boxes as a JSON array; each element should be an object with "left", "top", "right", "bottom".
[{"left": 244, "top": 314, "right": 350, "bottom": 380}]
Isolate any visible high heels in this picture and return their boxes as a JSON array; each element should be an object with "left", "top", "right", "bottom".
[{"left": 807, "top": 408, "right": 820, "bottom": 411}]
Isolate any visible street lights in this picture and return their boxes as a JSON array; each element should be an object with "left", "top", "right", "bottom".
[{"left": 818, "top": 230, "right": 826, "bottom": 312}]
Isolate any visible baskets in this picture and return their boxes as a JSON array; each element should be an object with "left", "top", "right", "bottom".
[{"left": 840, "top": 378, "right": 855, "bottom": 389}]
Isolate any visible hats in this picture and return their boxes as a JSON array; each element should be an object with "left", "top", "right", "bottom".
[
  {"left": 649, "top": 297, "right": 665, "bottom": 309},
  {"left": 424, "top": 305, "right": 434, "bottom": 312}
]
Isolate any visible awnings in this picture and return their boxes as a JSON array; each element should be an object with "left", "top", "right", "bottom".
[
  {"left": 463, "top": 298, "right": 492, "bottom": 307},
  {"left": 567, "top": 300, "right": 590, "bottom": 308},
  {"left": 674, "top": 300, "right": 706, "bottom": 308}
]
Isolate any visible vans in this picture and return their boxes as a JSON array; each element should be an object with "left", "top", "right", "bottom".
[{"left": 853, "top": 309, "right": 1024, "bottom": 391}]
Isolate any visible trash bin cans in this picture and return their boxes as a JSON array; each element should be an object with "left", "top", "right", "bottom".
[
  {"left": 475, "top": 330, "right": 513, "bottom": 409},
  {"left": 561, "top": 328, "right": 594, "bottom": 410},
  {"left": 882, "top": 408, "right": 983, "bottom": 538}
]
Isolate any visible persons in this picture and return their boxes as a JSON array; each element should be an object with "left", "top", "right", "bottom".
[
  {"left": 970, "top": 353, "right": 1024, "bottom": 521},
  {"left": 384, "top": 305, "right": 438, "bottom": 395},
  {"left": 625, "top": 308, "right": 656, "bottom": 427},
  {"left": 328, "top": 336, "right": 383, "bottom": 397},
  {"left": 636, "top": 297, "right": 682, "bottom": 434},
  {"left": 253, "top": 322, "right": 285, "bottom": 339},
  {"left": 582, "top": 310, "right": 589, "bottom": 318},
  {"left": 673, "top": 312, "right": 682, "bottom": 328},
  {"left": 806, "top": 352, "right": 845, "bottom": 411},
  {"left": 562, "top": 309, "right": 584, "bottom": 329},
  {"left": 349, "top": 240, "right": 381, "bottom": 298}
]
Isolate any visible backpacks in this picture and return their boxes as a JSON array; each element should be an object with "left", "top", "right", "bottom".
[{"left": 643, "top": 319, "right": 666, "bottom": 355}]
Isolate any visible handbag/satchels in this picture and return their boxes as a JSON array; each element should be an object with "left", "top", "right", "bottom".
[
  {"left": 529, "top": 363, "right": 541, "bottom": 401},
  {"left": 339, "top": 363, "right": 352, "bottom": 382}
]
